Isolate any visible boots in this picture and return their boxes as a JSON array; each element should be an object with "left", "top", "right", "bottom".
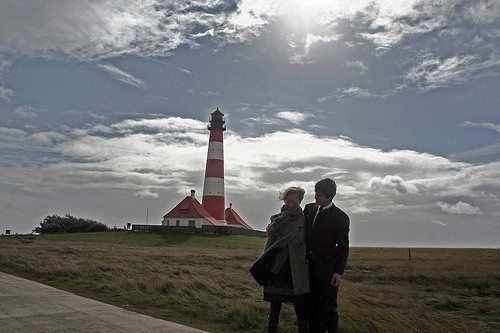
[
  {"left": 267, "top": 317, "right": 279, "bottom": 333},
  {"left": 296, "top": 320, "right": 308, "bottom": 333}
]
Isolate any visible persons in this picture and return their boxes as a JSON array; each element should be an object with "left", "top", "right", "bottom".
[
  {"left": 249, "top": 188, "right": 310, "bottom": 333},
  {"left": 304, "top": 178, "right": 349, "bottom": 333}
]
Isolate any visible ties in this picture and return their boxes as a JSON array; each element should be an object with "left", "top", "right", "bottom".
[{"left": 314, "top": 206, "right": 324, "bottom": 228}]
